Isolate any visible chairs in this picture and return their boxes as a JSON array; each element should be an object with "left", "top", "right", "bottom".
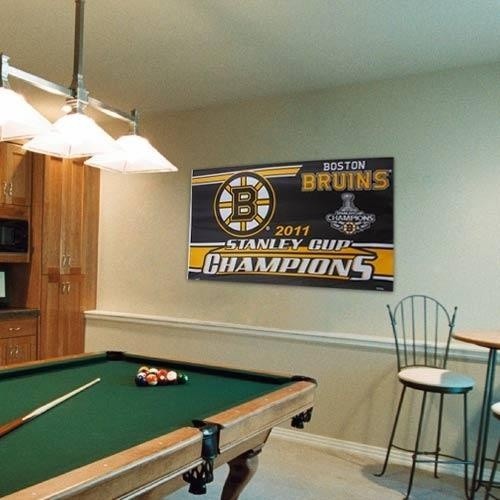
[
  {"left": 470, "top": 402, "right": 500, "bottom": 500},
  {"left": 372, "top": 295, "right": 476, "bottom": 500}
]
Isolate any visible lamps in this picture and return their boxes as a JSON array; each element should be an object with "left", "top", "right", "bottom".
[{"left": 0, "top": 0, "right": 179, "bottom": 173}]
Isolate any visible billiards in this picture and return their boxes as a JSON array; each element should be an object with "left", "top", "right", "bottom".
[{"left": 135, "top": 365, "right": 188, "bottom": 385}]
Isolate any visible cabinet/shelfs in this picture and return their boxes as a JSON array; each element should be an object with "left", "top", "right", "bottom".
[{"left": 0, "top": 141, "right": 100, "bottom": 367}]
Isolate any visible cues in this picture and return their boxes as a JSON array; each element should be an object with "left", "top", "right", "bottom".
[{"left": 1, "top": 378, "right": 101, "bottom": 435}]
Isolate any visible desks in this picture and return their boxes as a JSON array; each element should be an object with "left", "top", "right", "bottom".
[
  {"left": 0, "top": 350, "right": 317, "bottom": 500},
  {"left": 453, "top": 331, "right": 500, "bottom": 500}
]
